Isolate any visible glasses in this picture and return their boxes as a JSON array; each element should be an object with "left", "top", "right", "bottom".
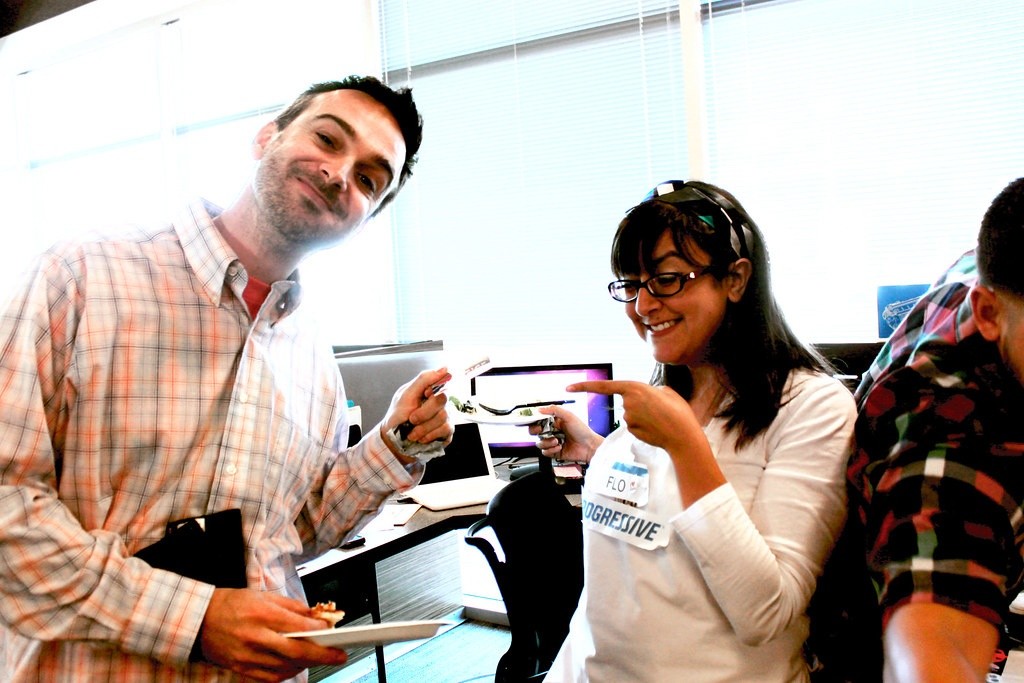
[{"left": 608, "top": 265, "right": 710, "bottom": 304}]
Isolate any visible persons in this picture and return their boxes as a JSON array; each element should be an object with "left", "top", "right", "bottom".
[
  {"left": 798, "top": 175, "right": 1024, "bottom": 683},
  {"left": 0, "top": 71, "right": 457, "bottom": 683},
  {"left": 524, "top": 178, "right": 861, "bottom": 683}
]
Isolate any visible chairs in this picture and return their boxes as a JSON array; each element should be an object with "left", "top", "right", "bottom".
[{"left": 460, "top": 470, "right": 585, "bottom": 683}]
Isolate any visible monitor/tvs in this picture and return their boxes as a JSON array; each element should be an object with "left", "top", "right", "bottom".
[{"left": 472, "top": 363, "right": 615, "bottom": 459}]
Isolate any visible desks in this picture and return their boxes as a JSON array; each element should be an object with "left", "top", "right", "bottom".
[{"left": 294, "top": 466, "right": 582, "bottom": 678}]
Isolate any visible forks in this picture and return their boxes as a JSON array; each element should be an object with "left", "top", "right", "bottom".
[{"left": 476, "top": 398, "right": 567, "bottom": 417}]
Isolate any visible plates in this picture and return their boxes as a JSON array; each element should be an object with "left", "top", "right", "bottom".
[
  {"left": 452, "top": 394, "right": 554, "bottom": 426},
  {"left": 282, "top": 618, "right": 456, "bottom": 651}
]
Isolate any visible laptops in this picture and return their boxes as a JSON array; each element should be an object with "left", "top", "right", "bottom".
[{"left": 408, "top": 421, "right": 508, "bottom": 513}]
